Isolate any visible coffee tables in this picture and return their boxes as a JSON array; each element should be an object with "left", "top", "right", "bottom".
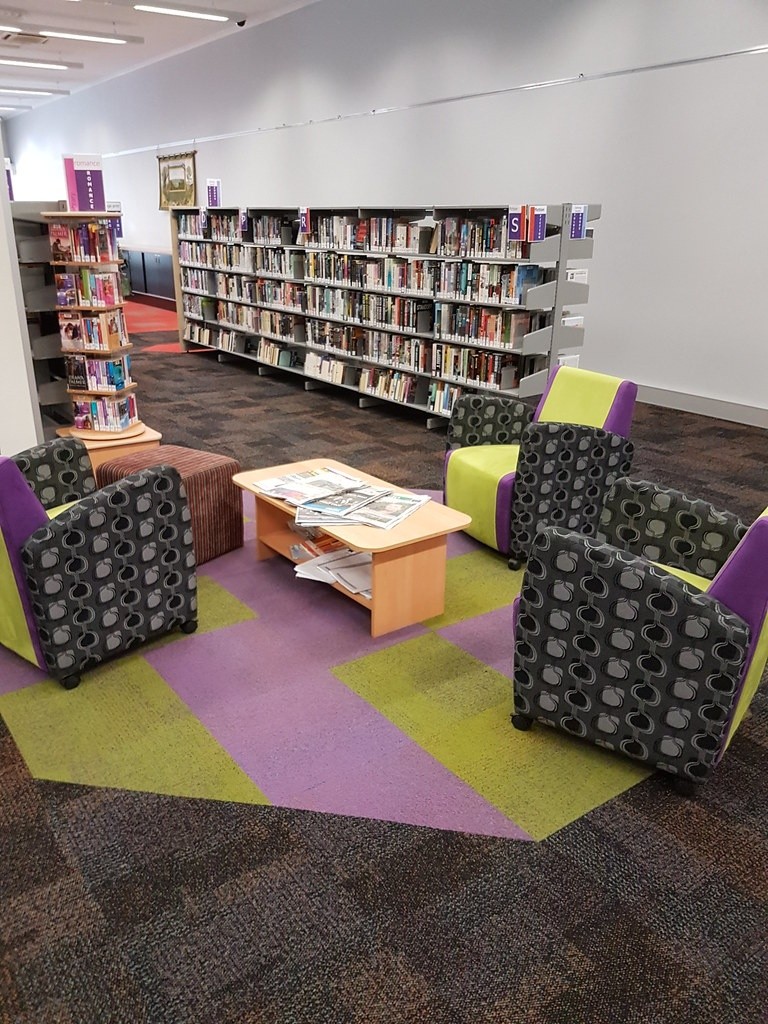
[{"left": 231, "top": 462, "right": 468, "bottom": 639}]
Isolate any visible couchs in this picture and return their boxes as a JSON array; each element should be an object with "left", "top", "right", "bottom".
[
  {"left": 512, "top": 473, "right": 768, "bottom": 800},
  {"left": 0, "top": 437, "right": 199, "bottom": 690},
  {"left": 443, "top": 365, "right": 637, "bottom": 573}
]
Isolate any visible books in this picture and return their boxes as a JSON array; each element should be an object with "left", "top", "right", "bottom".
[
  {"left": 178, "top": 242, "right": 543, "bottom": 308},
  {"left": 177, "top": 268, "right": 538, "bottom": 420},
  {"left": 175, "top": 210, "right": 528, "bottom": 259},
  {"left": 47, "top": 219, "right": 138, "bottom": 434}
]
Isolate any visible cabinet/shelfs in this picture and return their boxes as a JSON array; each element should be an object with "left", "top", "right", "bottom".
[
  {"left": 11, "top": 200, "right": 82, "bottom": 446},
  {"left": 169, "top": 203, "right": 601, "bottom": 429},
  {"left": 41, "top": 212, "right": 146, "bottom": 441}
]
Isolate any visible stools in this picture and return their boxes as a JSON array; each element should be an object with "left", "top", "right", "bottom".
[{"left": 97, "top": 444, "right": 245, "bottom": 566}]
